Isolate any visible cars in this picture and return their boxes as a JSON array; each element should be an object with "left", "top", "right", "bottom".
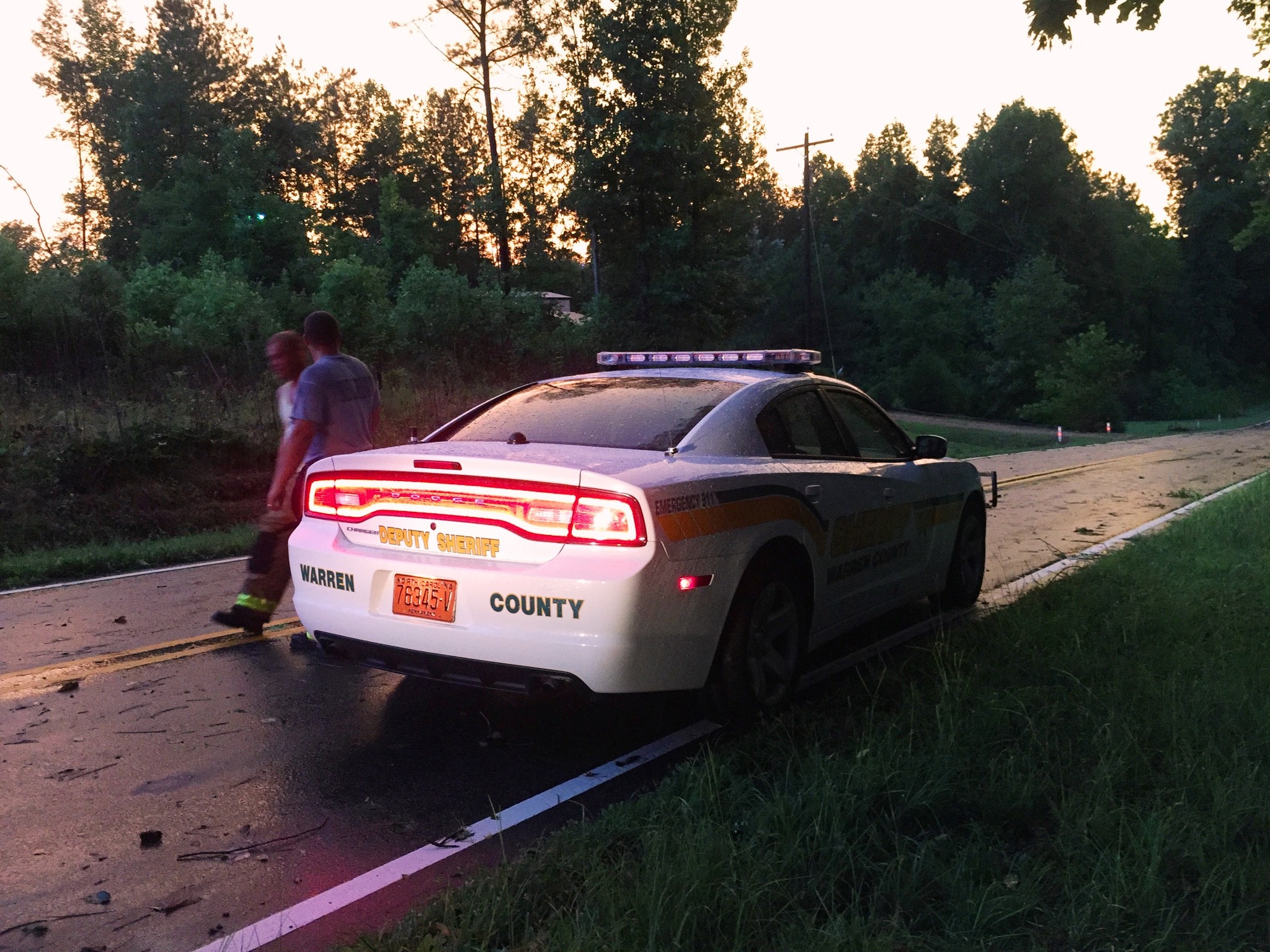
[{"left": 290, "top": 348, "right": 998, "bottom": 714}]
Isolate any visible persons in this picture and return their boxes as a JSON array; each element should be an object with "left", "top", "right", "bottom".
[
  {"left": 213, "top": 331, "right": 313, "bottom": 637},
  {"left": 265, "top": 311, "right": 389, "bottom": 669}
]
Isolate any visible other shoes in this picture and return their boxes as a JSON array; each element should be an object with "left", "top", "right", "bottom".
[
  {"left": 288, "top": 632, "right": 322, "bottom": 654},
  {"left": 210, "top": 604, "right": 263, "bottom": 636}
]
